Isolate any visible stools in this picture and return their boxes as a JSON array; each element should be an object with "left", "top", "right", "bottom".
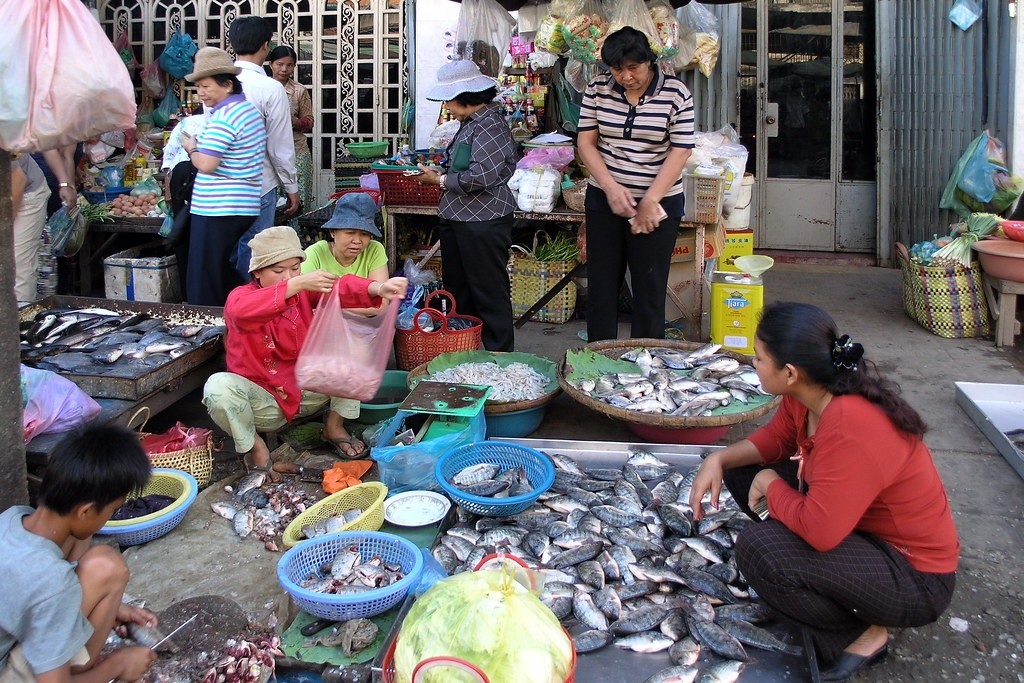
[{"left": 257, "top": 404, "right": 331, "bottom": 453}]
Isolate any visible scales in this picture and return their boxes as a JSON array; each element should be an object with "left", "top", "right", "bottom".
[{"left": 378, "top": 379, "right": 493, "bottom": 491}]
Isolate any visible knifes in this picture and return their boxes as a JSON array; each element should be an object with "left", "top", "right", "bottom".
[
  {"left": 107, "top": 613, "right": 198, "bottom": 683},
  {"left": 273, "top": 462, "right": 325, "bottom": 481}
]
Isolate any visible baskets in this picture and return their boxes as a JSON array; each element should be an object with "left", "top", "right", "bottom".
[
  {"left": 682, "top": 174, "right": 724, "bottom": 224},
  {"left": 126, "top": 407, "right": 214, "bottom": 486},
  {"left": 328, "top": 190, "right": 381, "bottom": 206},
  {"left": 382, "top": 553, "right": 576, "bottom": 683},
  {"left": 97, "top": 467, "right": 199, "bottom": 546},
  {"left": 371, "top": 147, "right": 447, "bottom": 206},
  {"left": 282, "top": 481, "right": 388, "bottom": 547},
  {"left": 511, "top": 258, "right": 577, "bottom": 324},
  {"left": 344, "top": 142, "right": 390, "bottom": 158},
  {"left": 563, "top": 178, "right": 587, "bottom": 211},
  {"left": 434, "top": 441, "right": 555, "bottom": 516},
  {"left": 276, "top": 530, "right": 423, "bottom": 621}
]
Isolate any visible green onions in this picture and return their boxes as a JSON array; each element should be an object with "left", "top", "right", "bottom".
[{"left": 523, "top": 231, "right": 581, "bottom": 261}]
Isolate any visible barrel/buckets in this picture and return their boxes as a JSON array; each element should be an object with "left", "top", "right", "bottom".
[{"left": 710, "top": 173, "right": 764, "bottom": 357}]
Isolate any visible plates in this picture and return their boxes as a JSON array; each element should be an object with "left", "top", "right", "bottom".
[{"left": 384, "top": 490, "right": 451, "bottom": 526}]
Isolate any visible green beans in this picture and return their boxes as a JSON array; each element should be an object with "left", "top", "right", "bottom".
[{"left": 82, "top": 202, "right": 115, "bottom": 222}]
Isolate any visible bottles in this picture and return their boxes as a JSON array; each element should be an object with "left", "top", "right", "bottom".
[
  {"left": 163, "top": 114, "right": 179, "bottom": 147},
  {"left": 388, "top": 144, "right": 442, "bottom": 167},
  {"left": 439, "top": 104, "right": 455, "bottom": 126},
  {"left": 502, "top": 61, "right": 540, "bottom": 93},
  {"left": 124, "top": 160, "right": 135, "bottom": 181},
  {"left": 136, "top": 154, "right": 146, "bottom": 181},
  {"left": 176, "top": 100, "right": 199, "bottom": 117},
  {"left": 526, "top": 99, "right": 540, "bottom": 139},
  {"left": 518, "top": 99, "right": 525, "bottom": 120},
  {"left": 502, "top": 99, "right": 512, "bottom": 129}
]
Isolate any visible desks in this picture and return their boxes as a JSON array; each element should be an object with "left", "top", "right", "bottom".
[{"left": 386, "top": 201, "right": 705, "bottom": 343}]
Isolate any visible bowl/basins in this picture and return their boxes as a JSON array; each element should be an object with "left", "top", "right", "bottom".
[
  {"left": 345, "top": 142, "right": 387, "bottom": 158},
  {"left": 157, "top": 198, "right": 173, "bottom": 216},
  {"left": 971, "top": 240, "right": 1024, "bottom": 282},
  {"left": 79, "top": 187, "right": 134, "bottom": 204},
  {"left": 357, "top": 370, "right": 411, "bottom": 424},
  {"left": 485, "top": 403, "right": 547, "bottom": 439}
]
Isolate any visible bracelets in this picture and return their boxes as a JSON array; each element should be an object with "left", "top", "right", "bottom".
[{"left": 58, "top": 182, "right": 75, "bottom": 190}]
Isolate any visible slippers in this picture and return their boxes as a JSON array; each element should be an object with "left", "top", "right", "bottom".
[
  {"left": 322, "top": 433, "right": 371, "bottom": 460},
  {"left": 243, "top": 453, "right": 283, "bottom": 484}
]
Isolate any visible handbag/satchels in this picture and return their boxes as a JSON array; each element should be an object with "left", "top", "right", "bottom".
[
  {"left": 293, "top": 277, "right": 401, "bottom": 402},
  {"left": 399, "top": 290, "right": 483, "bottom": 371},
  {"left": 157, "top": 207, "right": 175, "bottom": 237},
  {"left": 895, "top": 241, "right": 989, "bottom": 338},
  {"left": 451, "top": 143, "right": 471, "bottom": 173},
  {"left": 0, "top": 0, "right": 137, "bottom": 155},
  {"left": 81, "top": 26, "right": 199, "bottom": 196},
  {"left": 46, "top": 203, "right": 85, "bottom": 258},
  {"left": 454, "top": 0, "right": 723, "bottom": 94}
]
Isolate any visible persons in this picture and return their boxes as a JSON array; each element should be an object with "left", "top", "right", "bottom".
[
  {"left": 268, "top": 46, "right": 313, "bottom": 234},
  {"left": 180, "top": 45, "right": 266, "bottom": 306},
  {"left": 161, "top": 100, "right": 205, "bottom": 301},
  {"left": 576, "top": 26, "right": 695, "bottom": 344},
  {"left": 10, "top": 142, "right": 84, "bottom": 304},
  {"left": 202, "top": 225, "right": 407, "bottom": 485},
  {"left": 0, "top": 419, "right": 157, "bottom": 683},
  {"left": 231, "top": 16, "right": 300, "bottom": 280},
  {"left": 300, "top": 191, "right": 390, "bottom": 316},
  {"left": 413, "top": 59, "right": 519, "bottom": 353},
  {"left": 689, "top": 302, "right": 961, "bottom": 683}
]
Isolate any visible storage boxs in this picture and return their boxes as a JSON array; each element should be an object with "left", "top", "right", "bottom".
[
  {"left": 625, "top": 216, "right": 726, "bottom": 324},
  {"left": 298, "top": 205, "right": 384, "bottom": 251},
  {"left": 372, "top": 159, "right": 447, "bottom": 205},
  {"left": 682, "top": 164, "right": 726, "bottom": 224},
  {"left": 103, "top": 244, "right": 185, "bottom": 303}
]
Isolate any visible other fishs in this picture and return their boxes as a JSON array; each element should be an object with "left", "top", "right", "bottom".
[
  {"left": 419, "top": 359, "right": 551, "bottom": 402},
  {"left": 567, "top": 342, "right": 775, "bottom": 416},
  {"left": 300, "top": 503, "right": 366, "bottom": 540},
  {"left": 295, "top": 540, "right": 408, "bottom": 621},
  {"left": 123, "top": 597, "right": 183, "bottom": 656},
  {"left": 18, "top": 305, "right": 228, "bottom": 378},
  {"left": 431, "top": 445, "right": 805, "bottom": 683},
  {"left": 205, "top": 469, "right": 279, "bottom": 540}
]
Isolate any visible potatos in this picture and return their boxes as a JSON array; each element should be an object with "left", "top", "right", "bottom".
[{"left": 109, "top": 193, "right": 162, "bottom": 217}]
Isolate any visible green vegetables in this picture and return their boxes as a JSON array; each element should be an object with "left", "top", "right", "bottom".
[{"left": 928, "top": 216, "right": 998, "bottom": 268}]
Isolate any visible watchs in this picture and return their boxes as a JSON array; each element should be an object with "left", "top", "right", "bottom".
[{"left": 439, "top": 174, "right": 448, "bottom": 191}]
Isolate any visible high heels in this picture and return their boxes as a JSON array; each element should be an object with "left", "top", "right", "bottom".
[{"left": 819, "top": 644, "right": 888, "bottom": 683}]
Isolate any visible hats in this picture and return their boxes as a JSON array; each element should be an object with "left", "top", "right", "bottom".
[
  {"left": 426, "top": 59, "right": 497, "bottom": 101},
  {"left": 320, "top": 193, "right": 382, "bottom": 238},
  {"left": 183, "top": 46, "right": 242, "bottom": 82},
  {"left": 246, "top": 226, "right": 307, "bottom": 273}
]
